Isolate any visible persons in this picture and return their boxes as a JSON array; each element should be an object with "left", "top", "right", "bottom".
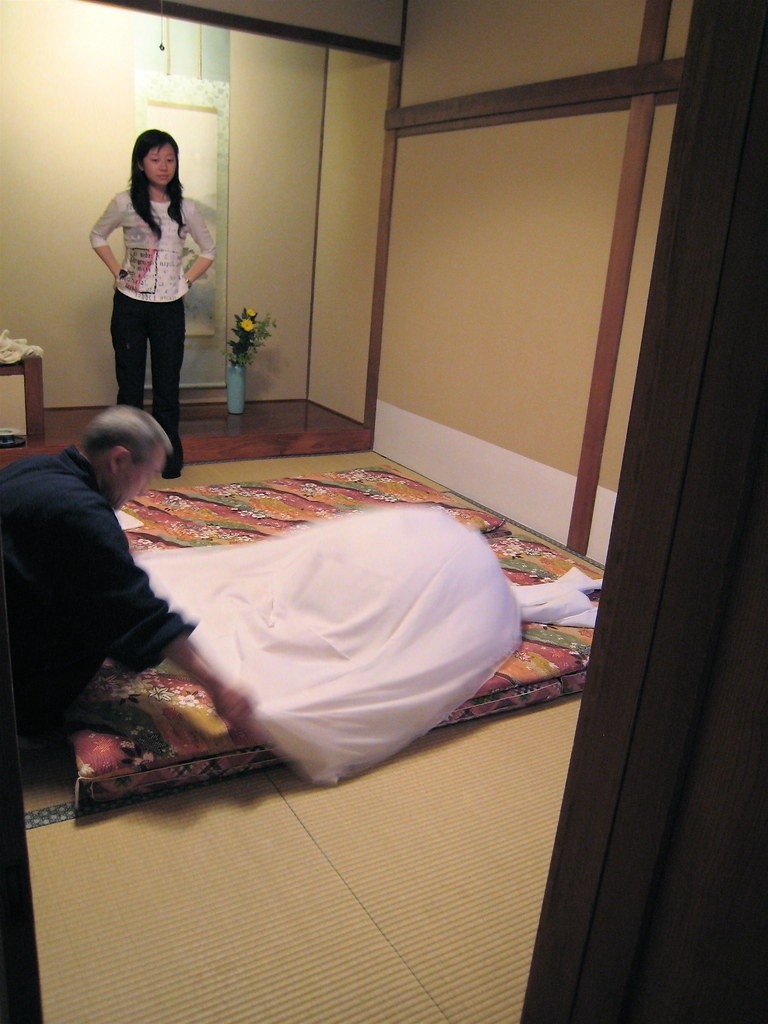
[
  {"left": 91, "top": 130, "right": 218, "bottom": 479},
  {"left": 0, "top": 405, "right": 253, "bottom": 787}
]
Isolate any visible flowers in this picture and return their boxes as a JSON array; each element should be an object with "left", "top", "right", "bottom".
[{"left": 218, "top": 307, "right": 277, "bottom": 367}]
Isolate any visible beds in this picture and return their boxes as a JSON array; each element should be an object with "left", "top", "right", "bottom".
[{"left": 55, "top": 465, "right": 600, "bottom": 812}]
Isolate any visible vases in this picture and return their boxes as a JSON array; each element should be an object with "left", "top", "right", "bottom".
[{"left": 227, "top": 363, "right": 246, "bottom": 415}]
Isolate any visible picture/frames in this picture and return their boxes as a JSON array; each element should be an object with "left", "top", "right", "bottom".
[{"left": 133, "top": 70, "right": 229, "bottom": 389}]
